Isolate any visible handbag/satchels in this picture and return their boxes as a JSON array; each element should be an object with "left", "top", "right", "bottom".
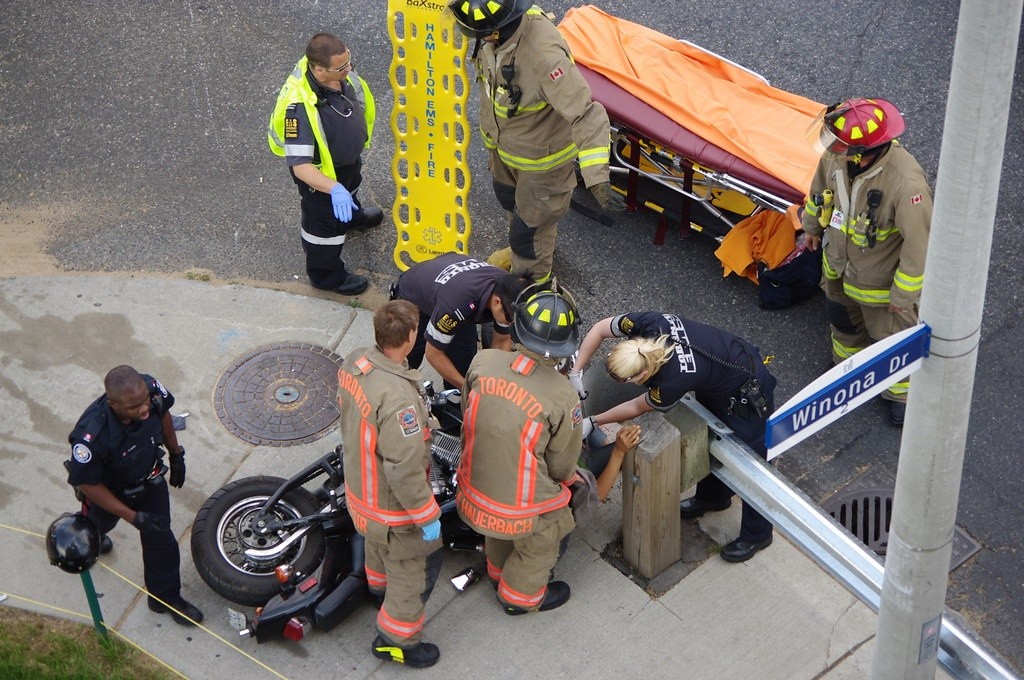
[{"left": 758, "top": 244, "right": 824, "bottom": 308}]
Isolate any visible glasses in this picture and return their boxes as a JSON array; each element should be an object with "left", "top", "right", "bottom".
[
  {"left": 604, "top": 353, "right": 642, "bottom": 383},
  {"left": 309, "top": 50, "right": 351, "bottom": 72}
]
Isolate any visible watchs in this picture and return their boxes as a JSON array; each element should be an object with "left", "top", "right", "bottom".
[{"left": 170, "top": 446, "right": 185, "bottom": 457}]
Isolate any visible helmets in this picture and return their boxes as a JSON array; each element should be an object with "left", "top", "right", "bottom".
[
  {"left": 449, "top": 0, "right": 535, "bottom": 38},
  {"left": 513, "top": 290, "right": 579, "bottom": 357},
  {"left": 820, "top": 98, "right": 907, "bottom": 156}
]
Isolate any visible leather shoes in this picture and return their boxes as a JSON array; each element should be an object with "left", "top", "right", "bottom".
[
  {"left": 349, "top": 207, "right": 383, "bottom": 229},
  {"left": 310, "top": 268, "right": 368, "bottom": 295},
  {"left": 148, "top": 594, "right": 203, "bottom": 626},
  {"left": 680, "top": 496, "right": 732, "bottom": 518},
  {"left": 100, "top": 535, "right": 112, "bottom": 554},
  {"left": 720, "top": 534, "right": 773, "bottom": 562},
  {"left": 506, "top": 581, "right": 570, "bottom": 615},
  {"left": 371, "top": 634, "right": 439, "bottom": 667}
]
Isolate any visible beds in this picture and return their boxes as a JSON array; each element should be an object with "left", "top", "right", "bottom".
[{"left": 547, "top": 4, "right": 832, "bottom": 308}]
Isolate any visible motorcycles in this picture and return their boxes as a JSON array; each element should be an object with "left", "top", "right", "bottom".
[{"left": 189, "top": 377, "right": 492, "bottom": 642}]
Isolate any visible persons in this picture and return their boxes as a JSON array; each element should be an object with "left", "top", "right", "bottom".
[
  {"left": 455, "top": 280, "right": 642, "bottom": 615},
  {"left": 64, "top": 364, "right": 203, "bottom": 626},
  {"left": 448, "top": 0, "right": 627, "bottom": 282},
  {"left": 569, "top": 311, "right": 777, "bottom": 563},
  {"left": 267, "top": 34, "right": 385, "bottom": 296},
  {"left": 388, "top": 250, "right": 536, "bottom": 392},
  {"left": 335, "top": 301, "right": 444, "bottom": 667},
  {"left": 802, "top": 96, "right": 933, "bottom": 428}
]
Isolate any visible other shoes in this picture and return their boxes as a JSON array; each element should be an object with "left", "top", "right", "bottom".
[
  {"left": 487, "top": 246, "right": 511, "bottom": 272},
  {"left": 890, "top": 400, "right": 906, "bottom": 425}
]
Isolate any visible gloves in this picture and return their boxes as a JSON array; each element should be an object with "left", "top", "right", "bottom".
[
  {"left": 590, "top": 182, "right": 627, "bottom": 214},
  {"left": 330, "top": 182, "right": 358, "bottom": 223},
  {"left": 169, "top": 445, "right": 185, "bottom": 488},
  {"left": 133, "top": 513, "right": 166, "bottom": 533},
  {"left": 581, "top": 416, "right": 593, "bottom": 441},
  {"left": 569, "top": 368, "right": 586, "bottom": 398}
]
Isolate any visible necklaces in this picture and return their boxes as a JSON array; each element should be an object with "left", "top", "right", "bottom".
[{"left": 307, "top": 68, "right": 355, "bottom": 117}]
